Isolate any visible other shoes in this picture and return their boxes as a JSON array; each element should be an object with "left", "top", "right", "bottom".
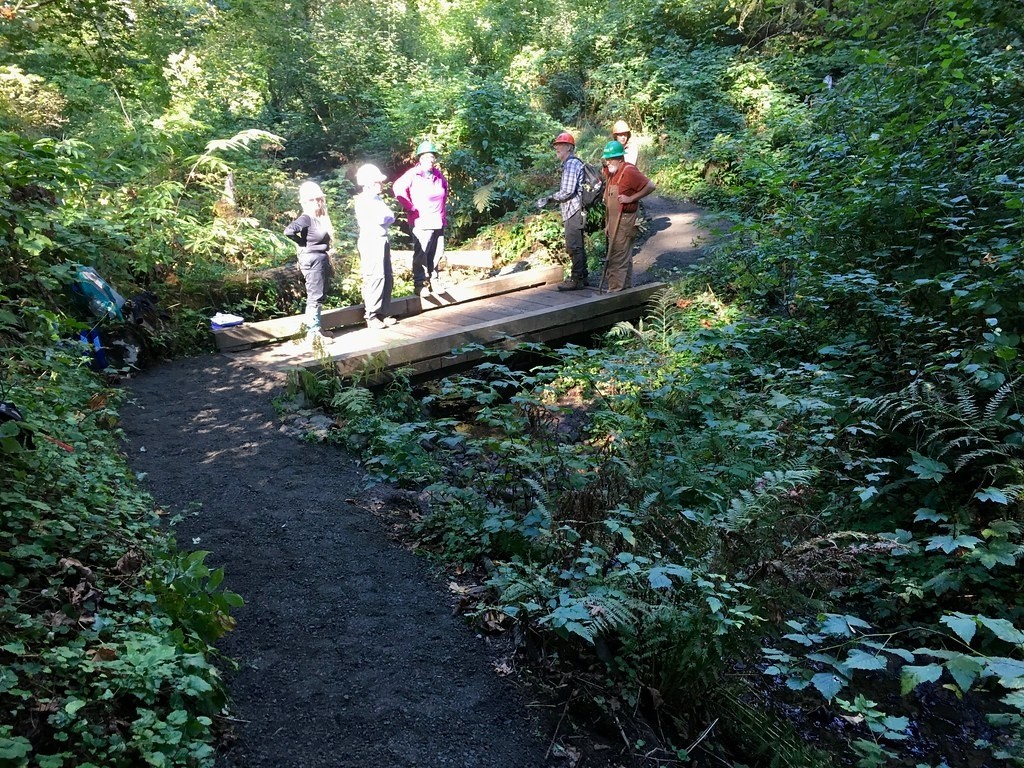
[
  {"left": 366, "top": 318, "right": 387, "bottom": 329},
  {"left": 557, "top": 278, "right": 589, "bottom": 291},
  {"left": 415, "top": 287, "right": 431, "bottom": 297},
  {"left": 430, "top": 282, "right": 446, "bottom": 294},
  {"left": 377, "top": 313, "right": 397, "bottom": 325},
  {"left": 307, "top": 331, "right": 333, "bottom": 344},
  {"left": 320, "top": 327, "right": 334, "bottom": 338}
]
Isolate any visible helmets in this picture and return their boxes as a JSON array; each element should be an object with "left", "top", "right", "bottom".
[
  {"left": 612, "top": 120, "right": 631, "bottom": 134},
  {"left": 553, "top": 133, "right": 575, "bottom": 146},
  {"left": 417, "top": 141, "right": 437, "bottom": 156},
  {"left": 601, "top": 140, "right": 628, "bottom": 159},
  {"left": 356, "top": 164, "right": 387, "bottom": 186},
  {"left": 298, "top": 181, "right": 324, "bottom": 203}
]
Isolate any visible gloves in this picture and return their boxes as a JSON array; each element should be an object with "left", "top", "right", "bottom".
[
  {"left": 538, "top": 194, "right": 554, "bottom": 208},
  {"left": 298, "top": 238, "right": 306, "bottom": 246}
]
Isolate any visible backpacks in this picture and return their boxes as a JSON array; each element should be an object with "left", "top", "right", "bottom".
[{"left": 566, "top": 156, "right": 603, "bottom": 205}]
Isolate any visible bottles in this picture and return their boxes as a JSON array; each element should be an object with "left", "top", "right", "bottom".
[{"left": 592, "top": 181, "right": 602, "bottom": 192}]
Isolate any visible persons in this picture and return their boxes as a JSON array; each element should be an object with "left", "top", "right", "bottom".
[
  {"left": 611, "top": 120, "right": 639, "bottom": 165},
  {"left": 283, "top": 181, "right": 336, "bottom": 347},
  {"left": 536, "top": 133, "right": 588, "bottom": 290},
  {"left": 355, "top": 164, "right": 395, "bottom": 330},
  {"left": 600, "top": 141, "right": 656, "bottom": 292},
  {"left": 393, "top": 140, "right": 448, "bottom": 298}
]
